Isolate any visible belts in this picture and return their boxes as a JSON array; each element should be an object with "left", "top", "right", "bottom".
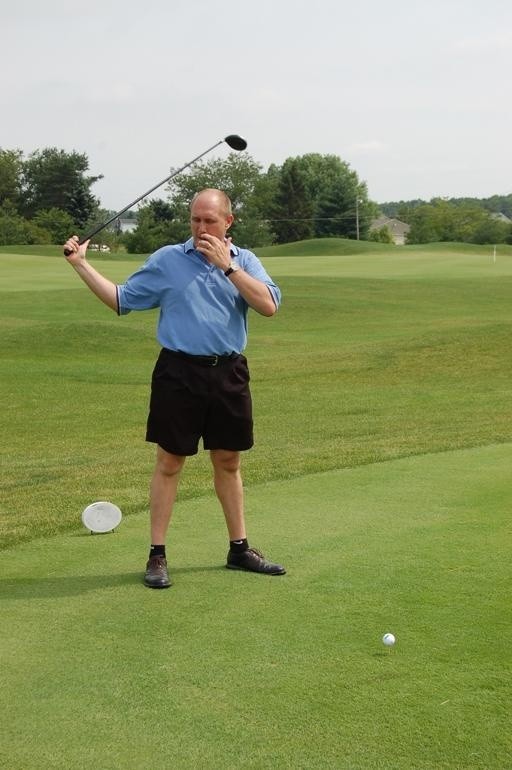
[{"left": 161, "top": 347, "right": 240, "bottom": 367}]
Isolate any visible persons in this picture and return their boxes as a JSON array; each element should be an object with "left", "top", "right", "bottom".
[{"left": 63, "top": 187, "right": 290, "bottom": 590}]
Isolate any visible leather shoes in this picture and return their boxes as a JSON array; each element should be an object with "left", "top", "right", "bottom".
[
  {"left": 143, "top": 554, "right": 171, "bottom": 588},
  {"left": 226, "top": 548, "right": 286, "bottom": 575}
]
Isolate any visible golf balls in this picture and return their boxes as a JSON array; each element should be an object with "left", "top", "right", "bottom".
[{"left": 382, "top": 632, "right": 396, "bottom": 646}]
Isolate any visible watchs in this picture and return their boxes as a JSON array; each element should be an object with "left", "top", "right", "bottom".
[{"left": 223, "top": 259, "right": 241, "bottom": 276}]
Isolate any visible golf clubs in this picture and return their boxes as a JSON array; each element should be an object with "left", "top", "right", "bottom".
[{"left": 64, "top": 134, "right": 246, "bottom": 256}]
